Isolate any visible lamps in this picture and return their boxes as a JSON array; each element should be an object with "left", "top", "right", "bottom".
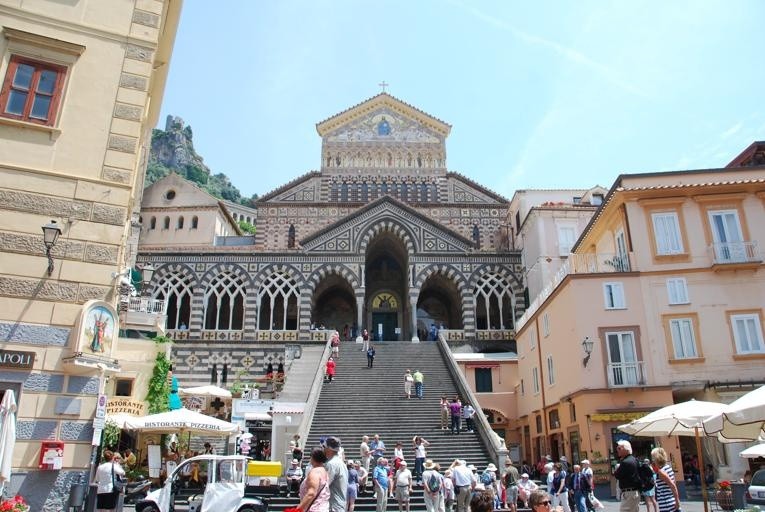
[{"left": 595, "top": 432, "right": 601, "bottom": 440}]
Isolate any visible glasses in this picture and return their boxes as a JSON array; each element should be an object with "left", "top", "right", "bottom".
[{"left": 539, "top": 499, "right": 552, "bottom": 506}]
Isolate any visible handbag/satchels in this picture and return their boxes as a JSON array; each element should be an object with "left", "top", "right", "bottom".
[{"left": 112, "top": 479, "right": 124, "bottom": 492}]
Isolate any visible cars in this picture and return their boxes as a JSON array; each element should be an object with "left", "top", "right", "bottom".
[
  {"left": 135, "top": 454, "right": 284, "bottom": 511},
  {"left": 743, "top": 469, "right": 765, "bottom": 512}
]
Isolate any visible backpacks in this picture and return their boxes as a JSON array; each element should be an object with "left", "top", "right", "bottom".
[{"left": 639, "top": 465, "right": 657, "bottom": 493}]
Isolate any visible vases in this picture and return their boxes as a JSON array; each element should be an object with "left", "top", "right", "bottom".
[{"left": 717, "top": 491, "right": 735, "bottom": 511}]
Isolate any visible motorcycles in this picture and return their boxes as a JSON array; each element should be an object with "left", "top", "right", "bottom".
[{"left": 123, "top": 472, "right": 153, "bottom": 505}]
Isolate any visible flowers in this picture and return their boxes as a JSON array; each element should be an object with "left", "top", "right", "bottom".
[{"left": 719, "top": 481, "right": 732, "bottom": 493}]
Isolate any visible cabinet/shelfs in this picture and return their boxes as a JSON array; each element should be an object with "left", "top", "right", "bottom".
[{"left": 590, "top": 459, "right": 610, "bottom": 486}]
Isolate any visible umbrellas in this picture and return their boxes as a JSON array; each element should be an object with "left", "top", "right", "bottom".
[
  {"left": 700, "top": 384, "right": 765, "bottom": 445},
  {"left": 103, "top": 412, "right": 136, "bottom": 453},
  {"left": 124, "top": 404, "right": 242, "bottom": 456},
  {"left": 0, "top": 386, "right": 18, "bottom": 491},
  {"left": 612, "top": 396, "right": 765, "bottom": 511},
  {"left": 178, "top": 384, "right": 233, "bottom": 415},
  {"left": 738, "top": 442, "right": 765, "bottom": 459}
]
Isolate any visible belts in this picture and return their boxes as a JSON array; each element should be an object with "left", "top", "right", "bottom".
[{"left": 619, "top": 488, "right": 639, "bottom": 492}]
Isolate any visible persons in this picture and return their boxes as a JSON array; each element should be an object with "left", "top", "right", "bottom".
[
  {"left": 329, "top": 334, "right": 341, "bottom": 359},
  {"left": 93, "top": 450, "right": 126, "bottom": 512},
  {"left": 413, "top": 369, "right": 425, "bottom": 399},
  {"left": 332, "top": 330, "right": 340, "bottom": 340},
  {"left": 366, "top": 344, "right": 375, "bottom": 369},
  {"left": 463, "top": 400, "right": 476, "bottom": 433},
  {"left": 417, "top": 321, "right": 449, "bottom": 341},
  {"left": 744, "top": 469, "right": 754, "bottom": 498},
  {"left": 325, "top": 357, "right": 336, "bottom": 384},
  {"left": 377, "top": 115, "right": 391, "bottom": 135},
  {"left": 448, "top": 398, "right": 462, "bottom": 435},
  {"left": 310, "top": 318, "right": 358, "bottom": 340},
  {"left": 404, "top": 368, "right": 413, "bottom": 399},
  {"left": 439, "top": 394, "right": 450, "bottom": 430},
  {"left": 453, "top": 394, "right": 463, "bottom": 430},
  {"left": 179, "top": 321, "right": 186, "bottom": 330},
  {"left": 361, "top": 328, "right": 369, "bottom": 352},
  {"left": 324, "top": 357, "right": 335, "bottom": 381}
]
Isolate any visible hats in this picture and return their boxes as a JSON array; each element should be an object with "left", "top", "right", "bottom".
[
  {"left": 422, "top": 459, "right": 437, "bottom": 469},
  {"left": 353, "top": 460, "right": 361, "bottom": 466},
  {"left": 642, "top": 458, "right": 650, "bottom": 465},
  {"left": 467, "top": 464, "right": 478, "bottom": 470},
  {"left": 291, "top": 459, "right": 300, "bottom": 465},
  {"left": 504, "top": 458, "right": 513, "bottom": 464},
  {"left": 486, "top": 462, "right": 498, "bottom": 471},
  {"left": 397, "top": 460, "right": 408, "bottom": 465},
  {"left": 520, "top": 453, "right": 592, "bottom": 478},
  {"left": 347, "top": 459, "right": 353, "bottom": 465},
  {"left": 321, "top": 436, "right": 341, "bottom": 452}
]
